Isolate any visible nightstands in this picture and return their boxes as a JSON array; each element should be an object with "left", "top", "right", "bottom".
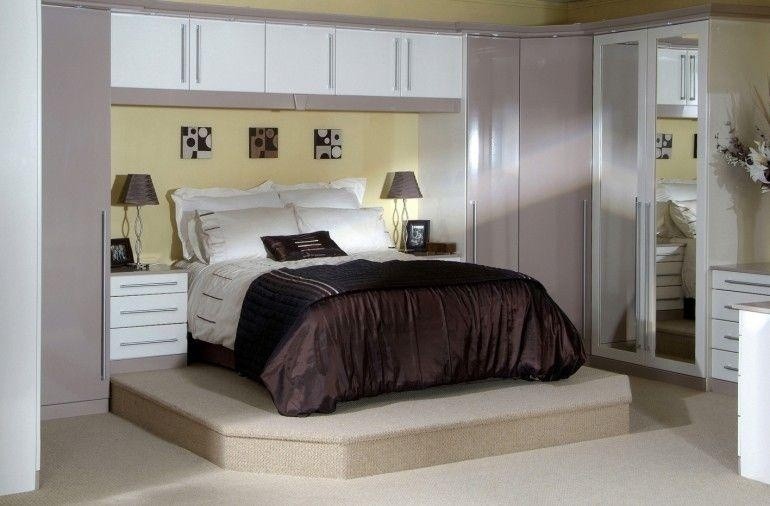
[
  {"left": 108, "top": 262, "right": 189, "bottom": 375},
  {"left": 656, "top": 236, "right": 686, "bottom": 318}
]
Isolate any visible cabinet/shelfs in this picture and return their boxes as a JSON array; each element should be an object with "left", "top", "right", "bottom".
[
  {"left": 733, "top": 301, "right": 769, "bottom": 486},
  {"left": 467, "top": 32, "right": 520, "bottom": 274},
  {"left": 335, "top": 29, "right": 461, "bottom": 115},
  {"left": 41, "top": 3, "right": 113, "bottom": 424},
  {"left": 519, "top": 34, "right": 593, "bottom": 357},
  {"left": 264, "top": 23, "right": 334, "bottom": 109},
  {"left": 111, "top": 11, "right": 266, "bottom": 107},
  {"left": 708, "top": 262, "right": 770, "bottom": 385},
  {"left": 601, "top": 40, "right": 642, "bottom": 357},
  {"left": 597, "top": 21, "right": 709, "bottom": 369}
]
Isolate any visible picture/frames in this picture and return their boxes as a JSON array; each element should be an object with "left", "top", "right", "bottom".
[
  {"left": 111, "top": 237, "right": 134, "bottom": 269},
  {"left": 405, "top": 219, "right": 430, "bottom": 249}
]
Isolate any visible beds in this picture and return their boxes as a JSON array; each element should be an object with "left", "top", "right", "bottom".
[
  {"left": 655, "top": 178, "right": 697, "bottom": 301},
  {"left": 172, "top": 252, "right": 585, "bottom": 417}
]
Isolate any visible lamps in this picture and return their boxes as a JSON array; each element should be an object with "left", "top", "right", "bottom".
[
  {"left": 379, "top": 170, "right": 423, "bottom": 253},
  {"left": 110, "top": 173, "right": 158, "bottom": 271}
]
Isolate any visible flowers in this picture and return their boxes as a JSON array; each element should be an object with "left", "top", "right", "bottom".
[{"left": 714, "top": 82, "right": 770, "bottom": 199}]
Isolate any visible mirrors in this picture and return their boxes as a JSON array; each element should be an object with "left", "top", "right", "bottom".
[{"left": 654, "top": 35, "right": 698, "bottom": 365}]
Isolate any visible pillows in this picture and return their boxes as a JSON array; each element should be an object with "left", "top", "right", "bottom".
[
  {"left": 260, "top": 230, "right": 347, "bottom": 264},
  {"left": 295, "top": 205, "right": 396, "bottom": 254},
  {"left": 272, "top": 177, "right": 366, "bottom": 212},
  {"left": 194, "top": 207, "right": 299, "bottom": 264},
  {"left": 667, "top": 199, "right": 697, "bottom": 239},
  {"left": 168, "top": 180, "right": 284, "bottom": 261}
]
